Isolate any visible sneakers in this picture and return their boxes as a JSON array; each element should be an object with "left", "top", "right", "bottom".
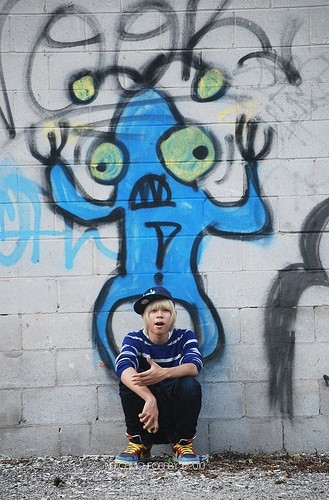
[
  {"left": 114, "top": 442, "right": 150, "bottom": 466},
  {"left": 172, "top": 438, "right": 201, "bottom": 465}
]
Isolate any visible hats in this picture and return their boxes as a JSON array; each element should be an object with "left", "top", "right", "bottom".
[{"left": 134, "top": 287, "right": 175, "bottom": 315}]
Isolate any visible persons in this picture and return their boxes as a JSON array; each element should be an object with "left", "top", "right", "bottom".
[{"left": 114, "top": 286, "right": 205, "bottom": 465}]
